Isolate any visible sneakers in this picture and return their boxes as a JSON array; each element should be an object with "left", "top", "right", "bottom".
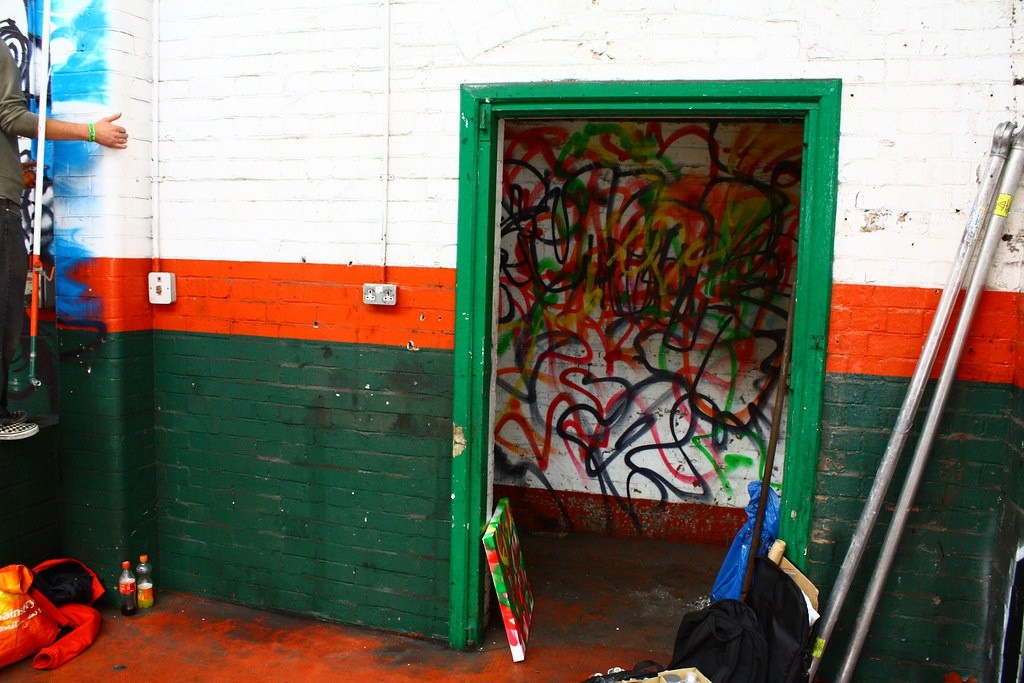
[
  {"left": 11, "top": 410, "right": 29, "bottom": 423},
  {"left": 0, "top": 420, "right": 39, "bottom": 440}
]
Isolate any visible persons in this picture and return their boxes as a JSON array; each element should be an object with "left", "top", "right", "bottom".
[{"left": 0, "top": 34, "right": 128, "bottom": 440}]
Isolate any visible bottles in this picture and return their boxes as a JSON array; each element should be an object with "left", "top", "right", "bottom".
[
  {"left": 136, "top": 555, "right": 154, "bottom": 609},
  {"left": 119, "top": 561, "right": 138, "bottom": 616}
]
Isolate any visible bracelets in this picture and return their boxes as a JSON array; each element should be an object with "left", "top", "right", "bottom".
[{"left": 89, "top": 123, "right": 95, "bottom": 142}]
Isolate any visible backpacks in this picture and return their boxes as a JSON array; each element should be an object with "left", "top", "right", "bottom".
[
  {"left": 742, "top": 556, "right": 821, "bottom": 683},
  {"left": 667, "top": 599, "right": 769, "bottom": 683}
]
